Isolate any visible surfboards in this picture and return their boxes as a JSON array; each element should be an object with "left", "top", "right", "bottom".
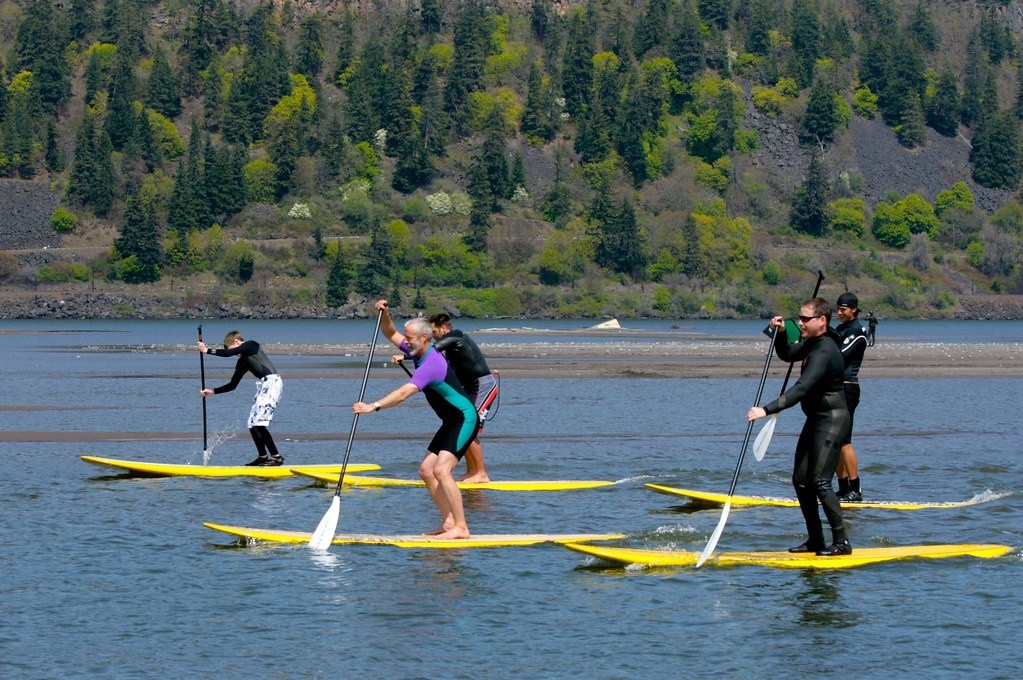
[
  {"left": 292, "top": 462, "right": 620, "bottom": 493},
  {"left": 202, "top": 518, "right": 632, "bottom": 553},
  {"left": 564, "top": 538, "right": 1016, "bottom": 570},
  {"left": 80, "top": 451, "right": 385, "bottom": 483},
  {"left": 646, "top": 481, "right": 980, "bottom": 514}
]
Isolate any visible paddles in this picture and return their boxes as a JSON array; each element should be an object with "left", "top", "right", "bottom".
[
  {"left": 751, "top": 268, "right": 828, "bottom": 461},
  {"left": 195, "top": 323, "right": 213, "bottom": 465},
  {"left": 696, "top": 315, "right": 785, "bottom": 570},
  {"left": 399, "top": 361, "right": 413, "bottom": 379},
  {"left": 308, "top": 302, "right": 388, "bottom": 551}
]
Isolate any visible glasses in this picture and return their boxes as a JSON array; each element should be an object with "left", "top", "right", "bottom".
[
  {"left": 223, "top": 344, "right": 231, "bottom": 349},
  {"left": 798, "top": 314, "right": 821, "bottom": 323}
]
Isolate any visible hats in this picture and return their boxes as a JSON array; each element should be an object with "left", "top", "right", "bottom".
[{"left": 836, "top": 293, "right": 863, "bottom": 313}]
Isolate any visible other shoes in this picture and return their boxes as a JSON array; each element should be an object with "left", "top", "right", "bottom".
[
  {"left": 820, "top": 538, "right": 852, "bottom": 555},
  {"left": 245, "top": 456, "right": 269, "bottom": 466},
  {"left": 258, "top": 454, "right": 283, "bottom": 465},
  {"left": 835, "top": 487, "right": 849, "bottom": 498},
  {"left": 839, "top": 490, "right": 863, "bottom": 501},
  {"left": 789, "top": 537, "right": 825, "bottom": 552}
]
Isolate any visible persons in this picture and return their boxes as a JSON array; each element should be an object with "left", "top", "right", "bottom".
[
  {"left": 352, "top": 300, "right": 480, "bottom": 540},
  {"left": 747, "top": 298, "right": 852, "bottom": 556},
  {"left": 835, "top": 292, "right": 868, "bottom": 502},
  {"left": 390, "top": 312, "right": 498, "bottom": 483},
  {"left": 864, "top": 311, "right": 878, "bottom": 347},
  {"left": 198, "top": 331, "right": 286, "bottom": 466}
]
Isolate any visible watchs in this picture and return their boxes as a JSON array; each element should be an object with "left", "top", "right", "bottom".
[{"left": 372, "top": 402, "right": 380, "bottom": 412}]
